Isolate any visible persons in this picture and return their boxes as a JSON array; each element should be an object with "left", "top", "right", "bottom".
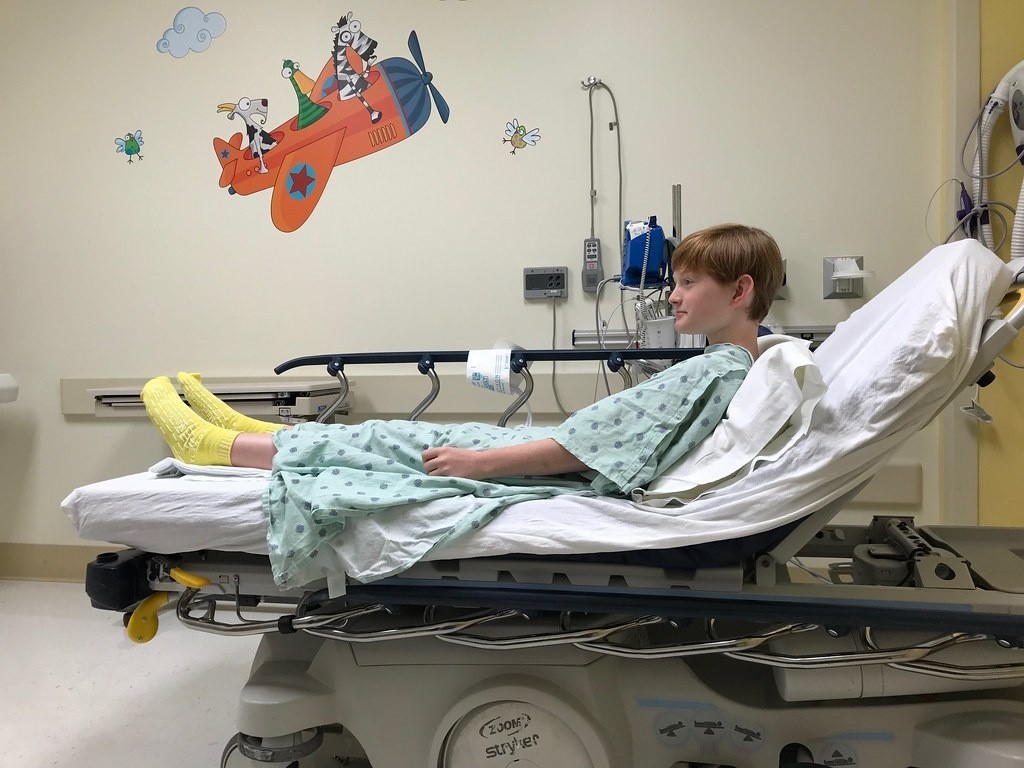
[{"left": 138, "top": 224, "right": 783, "bottom": 496}]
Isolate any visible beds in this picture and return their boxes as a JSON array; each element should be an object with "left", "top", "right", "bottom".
[{"left": 60, "top": 237, "right": 1024, "bottom": 768}]
